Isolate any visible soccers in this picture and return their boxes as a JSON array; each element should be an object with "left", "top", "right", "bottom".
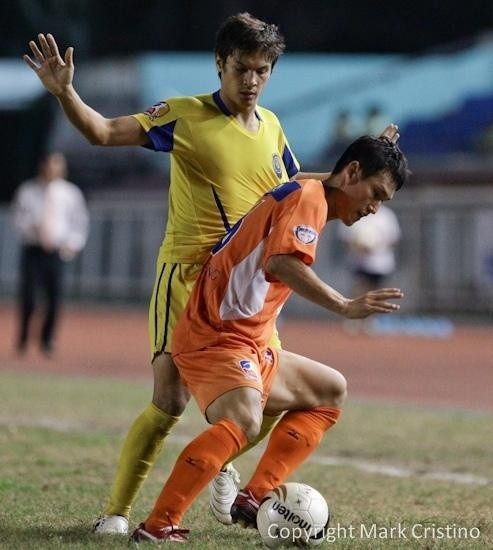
[{"left": 257, "top": 482, "right": 329, "bottom": 550}]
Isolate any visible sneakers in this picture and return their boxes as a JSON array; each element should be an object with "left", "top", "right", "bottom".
[
  {"left": 129, "top": 522, "right": 187, "bottom": 543},
  {"left": 210, "top": 462, "right": 242, "bottom": 524},
  {"left": 230, "top": 488, "right": 261, "bottom": 532},
  {"left": 91, "top": 513, "right": 129, "bottom": 535}
]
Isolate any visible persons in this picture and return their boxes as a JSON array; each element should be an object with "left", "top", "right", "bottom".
[
  {"left": 10, "top": 150, "right": 91, "bottom": 359},
  {"left": 361, "top": 104, "right": 388, "bottom": 141},
  {"left": 21, "top": 12, "right": 401, "bottom": 538},
  {"left": 124, "top": 134, "right": 412, "bottom": 546},
  {"left": 336, "top": 200, "right": 403, "bottom": 337},
  {"left": 321, "top": 109, "right": 356, "bottom": 171}
]
